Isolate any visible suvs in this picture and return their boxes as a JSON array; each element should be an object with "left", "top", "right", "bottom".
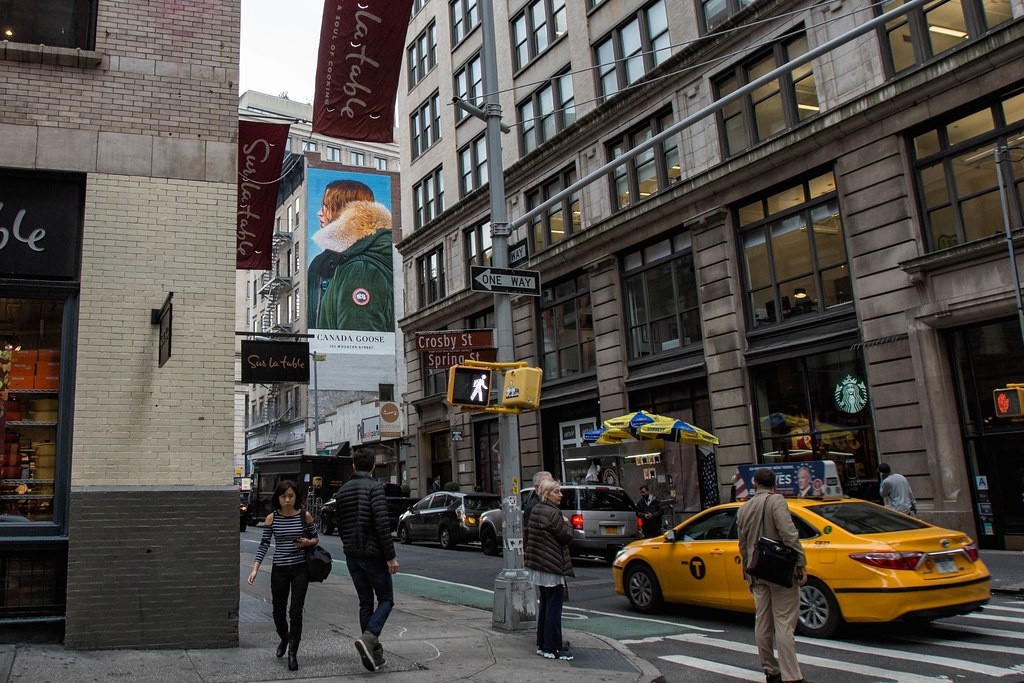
[{"left": 478, "top": 482, "right": 645, "bottom": 564}]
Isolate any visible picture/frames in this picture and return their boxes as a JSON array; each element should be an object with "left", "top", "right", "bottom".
[
  {"left": 641, "top": 322, "right": 661, "bottom": 343},
  {"left": 669, "top": 323, "right": 689, "bottom": 340}
]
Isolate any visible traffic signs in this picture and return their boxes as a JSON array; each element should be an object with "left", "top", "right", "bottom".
[{"left": 470, "top": 265, "right": 541, "bottom": 296}]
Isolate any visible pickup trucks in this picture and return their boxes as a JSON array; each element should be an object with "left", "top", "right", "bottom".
[{"left": 320, "top": 482, "right": 423, "bottom": 536}]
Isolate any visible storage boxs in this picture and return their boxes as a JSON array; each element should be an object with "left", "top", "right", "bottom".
[
  {"left": 8, "top": 349, "right": 61, "bottom": 390},
  {"left": 0, "top": 399, "right": 59, "bottom": 493}
]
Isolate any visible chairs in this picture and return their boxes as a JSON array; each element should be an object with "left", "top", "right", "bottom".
[
  {"left": 765, "top": 296, "right": 792, "bottom": 322},
  {"left": 835, "top": 275, "right": 853, "bottom": 305}
]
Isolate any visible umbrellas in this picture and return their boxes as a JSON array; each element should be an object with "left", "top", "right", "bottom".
[
  {"left": 582, "top": 428, "right": 633, "bottom": 445},
  {"left": 759, "top": 413, "right": 853, "bottom": 445},
  {"left": 601, "top": 408, "right": 676, "bottom": 440},
  {"left": 635, "top": 418, "right": 721, "bottom": 479}
]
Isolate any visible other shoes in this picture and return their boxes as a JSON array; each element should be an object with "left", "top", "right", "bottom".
[
  {"left": 288, "top": 651, "right": 299, "bottom": 671},
  {"left": 766, "top": 670, "right": 783, "bottom": 683},
  {"left": 275, "top": 636, "right": 289, "bottom": 658}
]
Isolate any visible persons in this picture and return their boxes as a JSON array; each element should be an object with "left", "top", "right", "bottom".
[
  {"left": 334, "top": 447, "right": 399, "bottom": 671},
  {"left": 797, "top": 466, "right": 816, "bottom": 496},
  {"left": 730, "top": 475, "right": 737, "bottom": 502},
  {"left": 521, "top": 471, "right": 576, "bottom": 660},
  {"left": 635, "top": 486, "right": 663, "bottom": 537},
  {"left": 247, "top": 480, "right": 319, "bottom": 672},
  {"left": 307, "top": 178, "right": 395, "bottom": 334},
  {"left": 734, "top": 467, "right": 811, "bottom": 683},
  {"left": 875, "top": 462, "right": 917, "bottom": 516},
  {"left": 431, "top": 475, "right": 441, "bottom": 492}
]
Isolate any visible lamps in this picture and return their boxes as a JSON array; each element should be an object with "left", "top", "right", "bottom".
[{"left": 794, "top": 288, "right": 806, "bottom": 298}]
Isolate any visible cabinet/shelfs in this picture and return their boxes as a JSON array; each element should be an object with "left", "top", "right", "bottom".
[{"left": 0, "top": 279, "right": 80, "bottom": 536}]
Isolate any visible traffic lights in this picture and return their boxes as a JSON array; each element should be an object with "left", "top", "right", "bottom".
[
  {"left": 993, "top": 388, "right": 1024, "bottom": 417},
  {"left": 446, "top": 365, "right": 493, "bottom": 407}
]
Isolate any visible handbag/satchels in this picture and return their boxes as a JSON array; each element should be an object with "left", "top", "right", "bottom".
[
  {"left": 745, "top": 535, "right": 798, "bottom": 588},
  {"left": 299, "top": 509, "right": 331, "bottom": 583}
]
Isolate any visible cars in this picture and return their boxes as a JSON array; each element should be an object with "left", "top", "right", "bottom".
[
  {"left": 612, "top": 494, "right": 993, "bottom": 640},
  {"left": 239, "top": 499, "right": 247, "bottom": 532},
  {"left": 397, "top": 490, "right": 502, "bottom": 549}
]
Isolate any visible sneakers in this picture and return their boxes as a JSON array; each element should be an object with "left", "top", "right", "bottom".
[
  {"left": 543, "top": 648, "right": 573, "bottom": 661},
  {"left": 536, "top": 645, "right": 546, "bottom": 654}
]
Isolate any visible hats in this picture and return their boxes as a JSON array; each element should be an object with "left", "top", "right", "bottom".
[
  {"left": 754, "top": 468, "right": 776, "bottom": 487},
  {"left": 876, "top": 463, "right": 890, "bottom": 474}
]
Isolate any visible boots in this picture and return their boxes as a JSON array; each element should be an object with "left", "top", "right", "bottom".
[
  {"left": 355, "top": 630, "right": 379, "bottom": 672},
  {"left": 372, "top": 643, "right": 386, "bottom": 670}
]
